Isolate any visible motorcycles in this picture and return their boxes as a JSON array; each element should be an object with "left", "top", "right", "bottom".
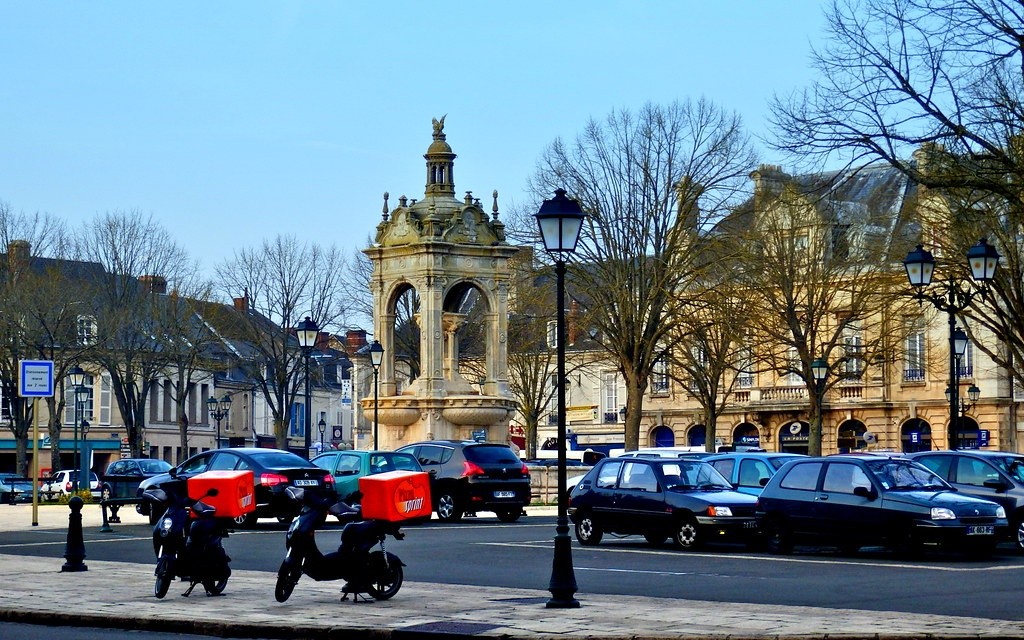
[
  {"left": 152, "top": 467, "right": 257, "bottom": 598},
  {"left": 274, "top": 469, "right": 431, "bottom": 603}
]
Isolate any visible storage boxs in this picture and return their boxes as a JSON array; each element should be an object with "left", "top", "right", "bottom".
[
  {"left": 187, "top": 470, "right": 256, "bottom": 518},
  {"left": 358, "top": 469, "right": 432, "bottom": 522}
]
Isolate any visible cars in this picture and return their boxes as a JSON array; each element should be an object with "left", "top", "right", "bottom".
[
  {"left": 276, "top": 449, "right": 425, "bottom": 526},
  {"left": 754, "top": 454, "right": 1009, "bottom": 563},
  {"left": 0, "top": 473, "right": 41, "bottom": 504},
  {"left": 101, "top": 459, "right": 174, "bottom": 503},
  {"left": 567, "top": 444, "right": 912, "bottom": 499},
  {"left": 375, "top": 440, "right": 531, "bottom": 523},
  {"left": 136, "top": 447, "right": 337, "bottom": 530},
  {"left": 40, "top": 469, "right": 102, "bottom": 502},
  {"left": 567, "top": 457, "right": 760, "bottom": 553},
  {"left": 901, "top": 451, "right": 1023, "bottom": 555}
]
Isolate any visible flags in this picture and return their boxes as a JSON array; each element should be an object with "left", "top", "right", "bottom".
[{"left": 338, "top": 378, "right": 353, "bottom": 412}]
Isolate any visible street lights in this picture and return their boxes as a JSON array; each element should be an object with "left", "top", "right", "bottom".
[
  {"left": 945, "top": 384, "right": 981, "bottom": 449},
  {"left": 318, "top": 419, "right": 326, "bottom": 454},
  {"left": 80, "top": 420, "right": 90, "bottom": 442},
  {"left": 206, "top": 395, "right": 233, "bottom": 448},
  {"left": 531, "top": 188, "right": 589, "bottom": 609},
  {"left": 294, "top": 316, "right": 321, "bottom": 448},
  {"left": 948, "top": 326, "right": 969, "bottom": 449},
  {"left": 904, "top": 238, "right": 1001, "bottom": 452},
  {"left": 76, "top": 385, "right": 90, "bottom": 441},
  {"left": 810, "top": 357, "right": 832, "bottom": 453},
  {"left": 68, "top": 359, "right": 86, "bottom": 497},
  {"left": 368, "top": 340, "right": 385, "bottom": 450}
]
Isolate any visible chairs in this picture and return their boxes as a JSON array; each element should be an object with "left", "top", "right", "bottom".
[
  {"left": 665, "top": 475, "right": 684, "bottom": 488},
  {"left": 629, "top": 473, "right": 650, "bottom": 484},
  {"left": 741, "top": 465, "right": 760, "bottom": 483},
  {"left": 341, "top": 465, "right": 353, "bottom": 474}
]
